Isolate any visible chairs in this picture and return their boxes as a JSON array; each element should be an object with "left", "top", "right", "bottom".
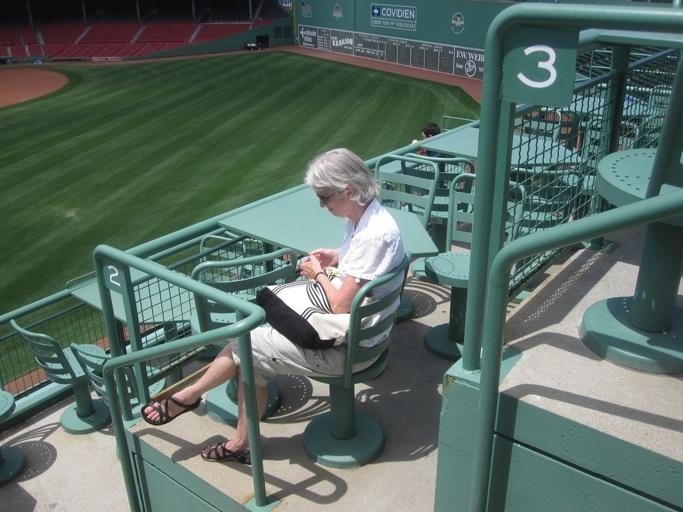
[
  {"left": 190, "top": 231, "right": 297, "bottom": 426},
  {"left": 302, "top": 253, "right": 413, "bottom": 470},
  {"left": 401, "top": 152, "right": 476, "bottom": 283},
  {"left": 69, "top": 342, "right": 166, "bottom": 417},
  {"left": 1, "top": 388, "right": 24, "bottom": 485},
  {"left": 10, "top": 319, "right": 112, "bottom": 435},
  {"left": 424, "top": 171, "right": 528, "bottom": 362},
  {"left": 579, "top": 53, "right": 683, "bottom": 376},
  {"left": 368, "top": 154, "right": 440, "bottom": 323},
  {"left": 507, "top": 69, "right": 670, "bottom": 271}
]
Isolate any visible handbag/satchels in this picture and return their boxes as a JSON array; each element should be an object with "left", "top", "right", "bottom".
[{"left": 256, "top": 269, "right": 371, "bottom": 349}]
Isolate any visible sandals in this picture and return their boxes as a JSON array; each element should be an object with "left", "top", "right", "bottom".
[
  {"left": 141, "top": 393, "right": 201, "bottom": 425},
  {"left": 200, "top": 440, "right": 251, "bottom": 465}
]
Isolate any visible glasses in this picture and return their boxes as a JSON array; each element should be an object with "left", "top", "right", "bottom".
[{"left": 317, "top": 192, "right": 336, "bottom": 203}]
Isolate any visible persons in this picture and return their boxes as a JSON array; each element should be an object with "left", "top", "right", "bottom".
[
  {"left": 410, "top": 122, "right": 460, "bottom": 174},
  {"left": 139, "top": 148, "right": 405, "bottom": 463}
]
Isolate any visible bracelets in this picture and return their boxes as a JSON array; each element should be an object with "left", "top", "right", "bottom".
[{"left": 314, "top": 271, "right": 324, "bottom": 280}]
